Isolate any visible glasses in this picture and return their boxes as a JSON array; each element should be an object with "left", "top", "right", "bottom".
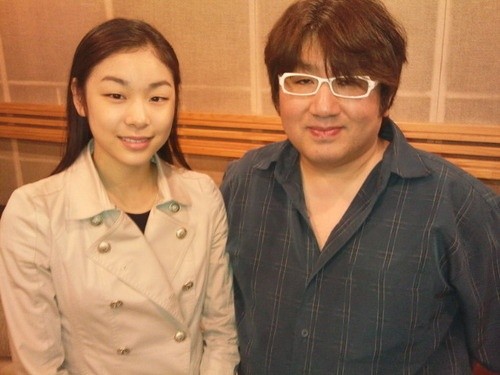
[{"left": 276, "top": 72, "right": 382, "bottom": 99}]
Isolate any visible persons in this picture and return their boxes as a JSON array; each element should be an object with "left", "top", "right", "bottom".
[
  {"left": 219, "top": 1, "right": 500, "bottom": 375},
  {"left": 1, "top": 17, "right": 241, "bottom": 375}
]
